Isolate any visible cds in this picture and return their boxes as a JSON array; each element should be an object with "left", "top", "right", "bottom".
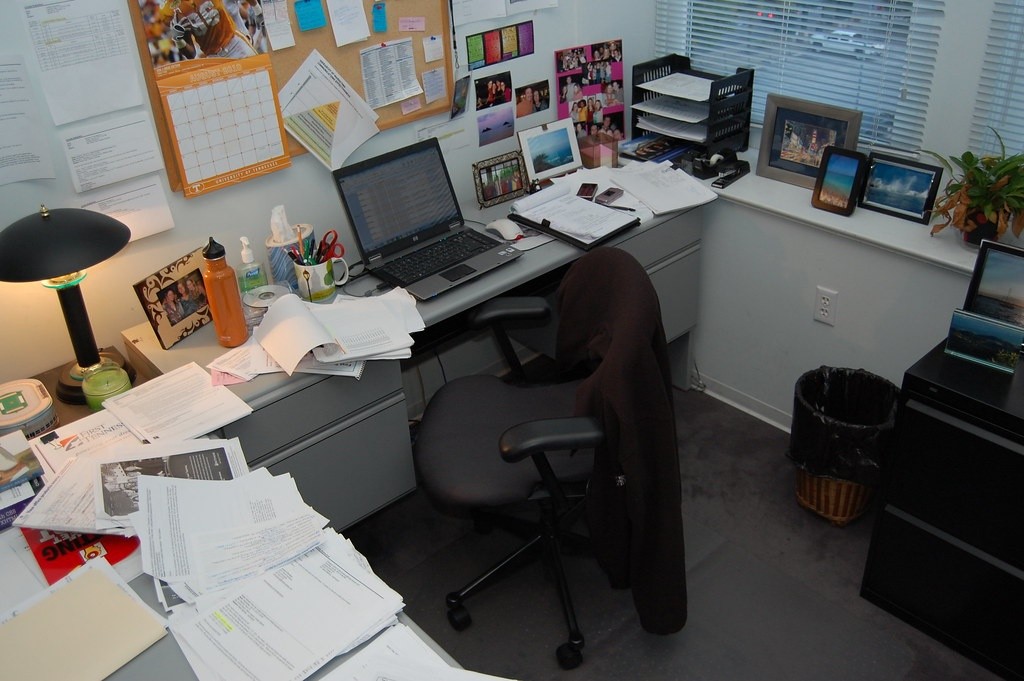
[{"left": 243, "top": 284, "right": 290, "bottom": 307}]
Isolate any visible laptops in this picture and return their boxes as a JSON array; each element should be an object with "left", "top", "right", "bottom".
[{"left": 332, "top": 137, "right": 525, "bottom": 302}]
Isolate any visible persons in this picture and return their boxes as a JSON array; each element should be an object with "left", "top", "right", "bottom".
[
  {"left": 168, "top": 0, "right": 265, "bottom": 61},
  {"left": 177, "top": 282, "right": 200, "bottom": 317},
  {"left": 476, "top": 80, "right": 512, "bottom": 110},
  {"left": 185, "top": 277, "right": 208, "bottom": 309},
  {"left": 516, "top": 87, "right": 535, "bottom": 118},
  {"left": 163, "top": 290, "right": 184, "bottom": 325},
  {"left": 532, "top": 91, "right": 548, "bottom": 112},
  {"left": 558, "top": 38, "right": 626, "bottom": 148}
]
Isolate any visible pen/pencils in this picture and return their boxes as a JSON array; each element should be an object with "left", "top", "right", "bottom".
[
  {"left": 297, "top": 224, "right": 304, "bottom": 256},
  {"left": 304, "top": 239, "right": 309, "bottom": 259},
  {"left": 317, "top": 239, "right": 330, "bottom": 260},
  {"left": 607, "top": 205, "right": 636, "bottom": 213},
  {"left": 291, "top": 246, "right": 302, "bottom": 261},
  {"left": 288, "top": 251, "right": 301, "bottom": 265},
  {"left": 310, "top": 238, "right": 315, "bottom": 257}
]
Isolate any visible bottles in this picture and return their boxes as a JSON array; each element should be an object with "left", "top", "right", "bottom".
[
  {"left": 82, "top": 362, "right": 132, "bottom": 411},
  {"left": 203, "top": 238, "right": 249, "bottom": 347}
]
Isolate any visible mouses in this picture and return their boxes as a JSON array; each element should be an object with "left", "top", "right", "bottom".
[{"left": 485, "top": 218, "right": 523, "bottom": 241}]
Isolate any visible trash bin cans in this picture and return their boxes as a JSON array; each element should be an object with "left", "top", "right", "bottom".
[{"left": 793, "top": 364, "right": 903, "bottom": 524}]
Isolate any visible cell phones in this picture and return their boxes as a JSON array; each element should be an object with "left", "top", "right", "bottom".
[
  {"left": 576, "top": 183, "right": 598, "bottom": 201},
  {"left": 595, "top": 187, "right": 623, "bottom": 205}
]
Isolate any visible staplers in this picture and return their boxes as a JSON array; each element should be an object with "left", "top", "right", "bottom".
[{"left": 711, "top": 160, "right": 751, "bottom": 189}]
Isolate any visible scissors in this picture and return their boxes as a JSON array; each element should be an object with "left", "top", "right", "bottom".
[{"left": 321, "top": 229, "right": 345, "bottom": 263}]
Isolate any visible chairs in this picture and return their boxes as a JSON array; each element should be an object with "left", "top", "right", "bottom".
[{"left": 415, "top": 246, "right": 685, "bottom": 671}]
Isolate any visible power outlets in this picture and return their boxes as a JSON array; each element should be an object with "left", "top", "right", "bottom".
[{"left": 812, "top": 285, "right": 839, "bottom": 326}]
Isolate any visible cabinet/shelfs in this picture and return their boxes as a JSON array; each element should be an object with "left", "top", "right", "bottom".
[
  {"left": 631, "top": 53, "right": 755, "bottom": 155},
  {"left": 858, "top": 335, "right": 1024, "bottom": 681}
]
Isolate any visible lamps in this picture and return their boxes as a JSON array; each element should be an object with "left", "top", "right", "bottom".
[{"left": 0, "top": 205, "right": 136, "bottom": 405}]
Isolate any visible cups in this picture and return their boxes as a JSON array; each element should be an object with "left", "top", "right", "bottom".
[{"left": 292, "top": 249, "right": 350, "bottom": 303}]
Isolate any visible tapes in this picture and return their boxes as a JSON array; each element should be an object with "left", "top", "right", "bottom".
[{"left": 708, "top": 154, "right": 725, "bottom": 165}]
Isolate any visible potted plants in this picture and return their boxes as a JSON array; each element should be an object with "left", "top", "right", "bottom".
[{"left": 915, "top": 126, "right": 1024, "bottom": 246}]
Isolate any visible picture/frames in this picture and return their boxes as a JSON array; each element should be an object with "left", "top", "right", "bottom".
[
  {"left": 473, "top": 150, "right": 531, "bottom": 210},
  {"left": 813, "top": 145, "right": 869, "bottom": 217},
  {"left": 755, "top": 92, "right": 864, "bottom": 191},
  {"left": 517, "top": 117, "right": 583, "bottom": 182},
  {"left": 129, "top": 248, "right": 224, "bottom": 350},
  {"left": 859, "top": 151, "right": 944, "bottom": 225}
]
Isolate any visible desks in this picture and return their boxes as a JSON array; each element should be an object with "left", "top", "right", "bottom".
[
  {"left": 0, "top": 344, "right": 525, "bottom": 681},
  {"left": 122, "top": 166, "right": 701, "bottom": 536}
]
